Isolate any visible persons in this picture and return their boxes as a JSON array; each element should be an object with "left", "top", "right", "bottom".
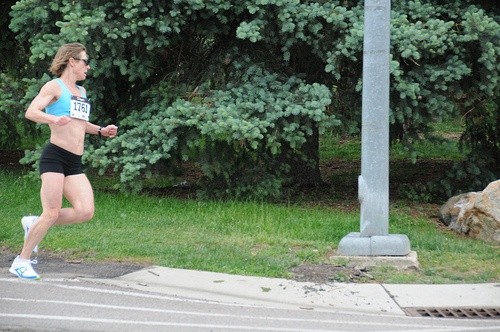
[{"left": 8, "top": 42, "right": 118, "bottom": 280}]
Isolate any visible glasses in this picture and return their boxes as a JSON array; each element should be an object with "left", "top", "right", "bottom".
[{"left": 73, "top": 57, "right": 90, "bottom": 65}]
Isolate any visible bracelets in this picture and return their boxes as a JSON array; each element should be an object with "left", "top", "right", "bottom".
[{"left": 98, "top": 126, "right": 103, "bottom": 138}]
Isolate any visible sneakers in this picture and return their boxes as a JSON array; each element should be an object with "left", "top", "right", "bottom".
[
  {"left": 9, "top": 255, "right": 40, "bottom": 281},
  {"left": 21, "top": 216, "right": 39, "bottom": 253}
]
